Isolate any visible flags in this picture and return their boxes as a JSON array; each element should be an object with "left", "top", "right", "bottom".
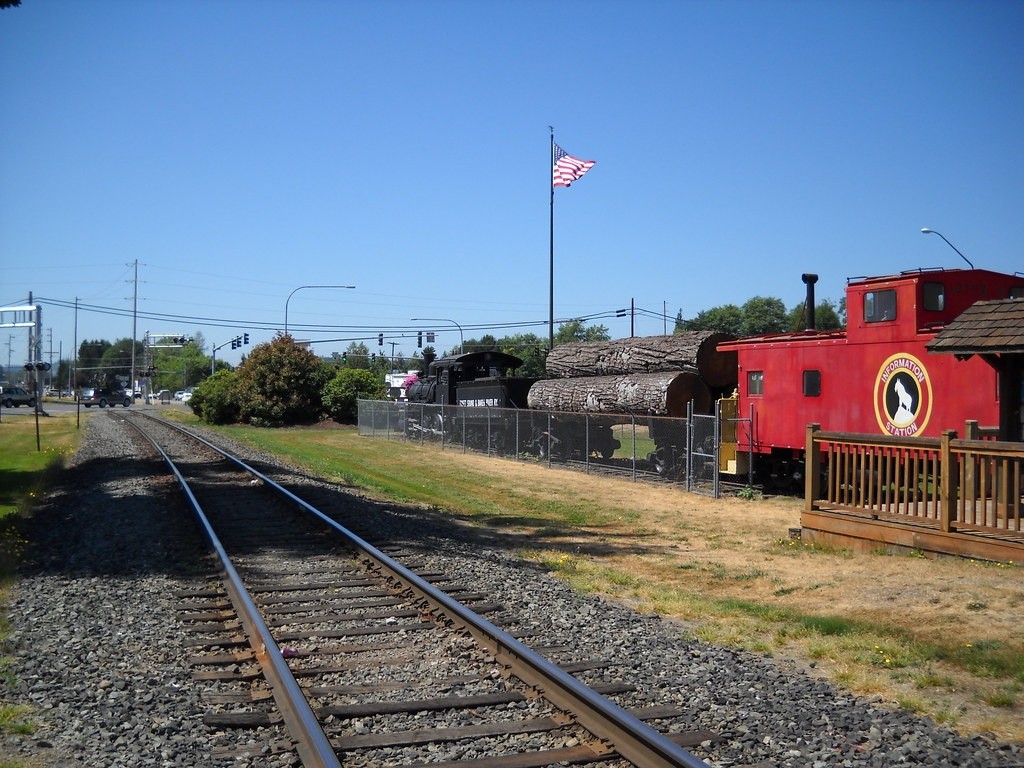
[{"left": 553, "top": 142, "right": 596, "bottom": 187}]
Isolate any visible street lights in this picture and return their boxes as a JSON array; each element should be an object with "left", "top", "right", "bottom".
[
  {"left": 410, "top": 317, "right": 464, "bottom": 354},
  {"left": 284, "top": 285, "right": 356, "bottom": 337}
]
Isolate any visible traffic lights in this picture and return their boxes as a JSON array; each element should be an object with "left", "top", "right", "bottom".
[
  {"left": 25, "top": 363, "right": 33, "bottom": 371},
  {"left": 243, "top": 333, "right": 249, "bottom": 344},
  {"left": 148, "top": 365, "right": 155, "bottom": 370},
  {"left": 145, "top": 372, "right": 150, "bottom": 377},
  {"left": 174, "top": 338, "right": 178, "bottom": 343},
  {"left": 140, "top": 372, "right": 145, "bottom": 377},
  {"left": 237, "top": 336, "right": 241, "bottom": 347},
  {"left": 179, "top": 338, "right": 184, "bottom": 343},
  {"left": 232, "top": 339, "right": 236, "bottom": 349},
  {"left": 342, "top": 351, "right": 346, "bottom": 363},
  {"left": 36, "top": 363, "right": 45, "bottom": 371},
  {"left": 372, "top": 352, "right": 375, "bottom": 362}
]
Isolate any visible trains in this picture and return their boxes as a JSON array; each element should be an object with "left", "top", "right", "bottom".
[{"left": 404, "top": 349, "right": 531, "bottom": 441}]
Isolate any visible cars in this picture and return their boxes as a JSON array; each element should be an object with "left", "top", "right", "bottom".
[{"left": 173, "top": 386, "right": 199, "bottom": 403}]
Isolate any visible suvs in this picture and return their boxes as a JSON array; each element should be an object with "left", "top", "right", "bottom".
[
  {"left": 46, "top": 388, "right": 67, "bottom": 397},
  {"left": 0, "top": 385, "right": 36, "bottom": 408},
  {"left": 79, "top": 387, "right": 131, "bottom": 408}
]
